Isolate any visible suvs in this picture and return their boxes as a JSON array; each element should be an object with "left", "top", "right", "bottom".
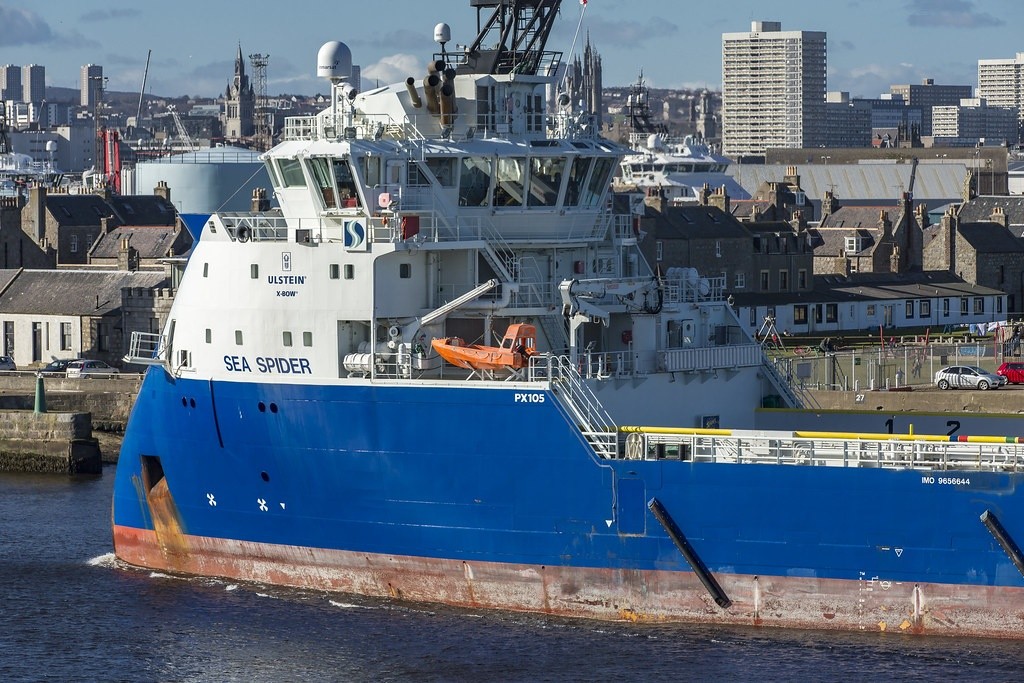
[{"left": 66, "top": 360, "right": 120, "bottom": 379}]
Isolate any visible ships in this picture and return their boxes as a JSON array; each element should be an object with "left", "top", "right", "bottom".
[{"left": 110, "top": 0, "right": 1024, "bottom": 639}]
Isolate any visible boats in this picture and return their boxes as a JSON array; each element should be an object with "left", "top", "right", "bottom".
[{"left": 431, "top": 324, "right": 540, "bottom": 371}]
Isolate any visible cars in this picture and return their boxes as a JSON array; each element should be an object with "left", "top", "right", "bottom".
[
  {"left": 934, "top": 365, "right": 1004, "bottom": 390},
  {"left": 0, "top": 356, "right": 16, "bottom": 370},
  {"left": 997, "top": 362, "right": 1024, "bottom": 385},
  {"left": 40, "top": 358, "right": 87, "bottom": 372}
]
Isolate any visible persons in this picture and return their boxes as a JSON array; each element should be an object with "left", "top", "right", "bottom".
[
  {"left": 897, "top": 367, "right": 905, "bottom": 384},
  {"left": 772, "top": 334, "right": 776, "bottom": 342},
  {"left": 913, "top": 358, "right": 921, "bottom": 378},
  {"left": 755, "top": 329, "right": 759, "bottom": 339},
  {"left": 783, "top": 329, "right": 790, "bottom": 336},
  {"left": 820, "top": 338, "right": 834, "bottom": 352},
  {"left": 836, "top": 336, "right": 844, "bottom": 344}
]
